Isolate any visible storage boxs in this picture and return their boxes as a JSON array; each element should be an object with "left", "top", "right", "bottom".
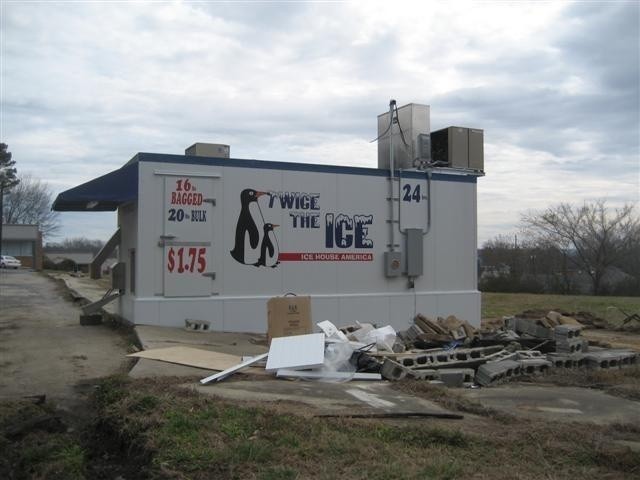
[{"left": 267, "top": 294, "right": 314, "bottom": 342}]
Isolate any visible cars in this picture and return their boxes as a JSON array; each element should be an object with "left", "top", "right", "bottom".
[{"left": 0, "top": 255, "right": 23, "bottom": 269}]
